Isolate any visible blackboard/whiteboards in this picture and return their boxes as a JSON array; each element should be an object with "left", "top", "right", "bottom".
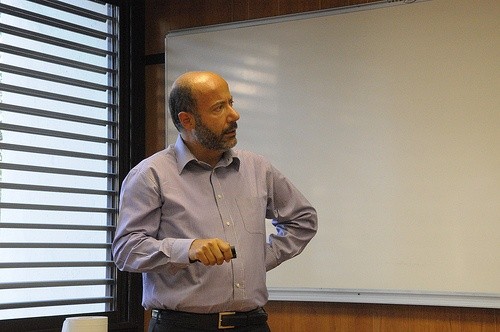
[{"left": 164, "top": 0, "right": 500, "bottom": 308}]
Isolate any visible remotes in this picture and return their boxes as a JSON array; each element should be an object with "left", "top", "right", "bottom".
[{"left": 220, "top": 246, "right": 237, "bottom": 260}]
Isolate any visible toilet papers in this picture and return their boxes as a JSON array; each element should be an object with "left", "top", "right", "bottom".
[{"left": 62, "top": 317, "right": 108, "bottom": 332}]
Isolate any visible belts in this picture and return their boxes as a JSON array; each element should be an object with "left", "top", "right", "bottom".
[{"left": 151, "top": 308, "right": 269, "bottom": 329}]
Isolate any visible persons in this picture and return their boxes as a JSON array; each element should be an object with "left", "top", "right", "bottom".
[{"left": 112, "top": 71, "right": 318, "bottom": 332}]
